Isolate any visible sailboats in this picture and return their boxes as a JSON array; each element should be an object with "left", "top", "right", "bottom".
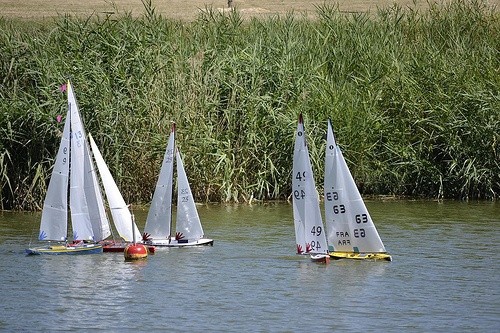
[
  {"left": 291, "top": 110, "right": 393, "bottom": 263},
  {"left": 24, "top": 71, "right": 215, "bottom": 255}
]
